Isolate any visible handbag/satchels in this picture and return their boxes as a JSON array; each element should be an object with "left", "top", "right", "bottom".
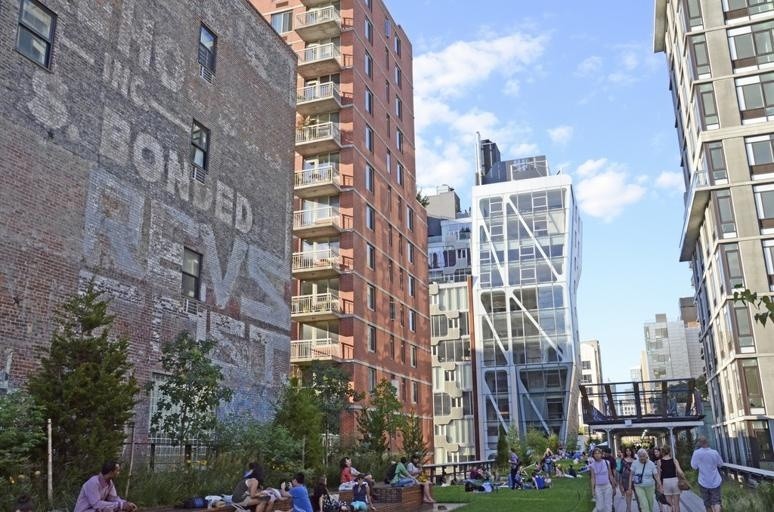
[
  {"left": 631, "top": 474, "right": 641, "bottom": 484},
  {"left": 678, "top": 478, "right": 689, "bottom": 489}
]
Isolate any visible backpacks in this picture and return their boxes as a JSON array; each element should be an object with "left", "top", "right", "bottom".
[{"left": 388, "top": 464, "right": 397, "bottom": 479}]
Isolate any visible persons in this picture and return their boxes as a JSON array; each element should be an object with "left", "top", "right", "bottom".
[
  {"left": 388, "top": 457, "right": 418, "bottom": 488},
  {"left": 231, "top": 452, "right": 377, "bottom": 511},
  {"left": 470, "top": 438, "right": 693, "bottom": 512},
  {"left": 691, "top": 436, "right": 724, "bottom": 512},
  {"left": 407, "top": 454, "right": 436, "bottom": 503},
  {"left": 73, "top": 460, "right": 138, "bottom": 512},
  {"left": 12, "top": 493, "right": 33, "bottom": 512}
]
